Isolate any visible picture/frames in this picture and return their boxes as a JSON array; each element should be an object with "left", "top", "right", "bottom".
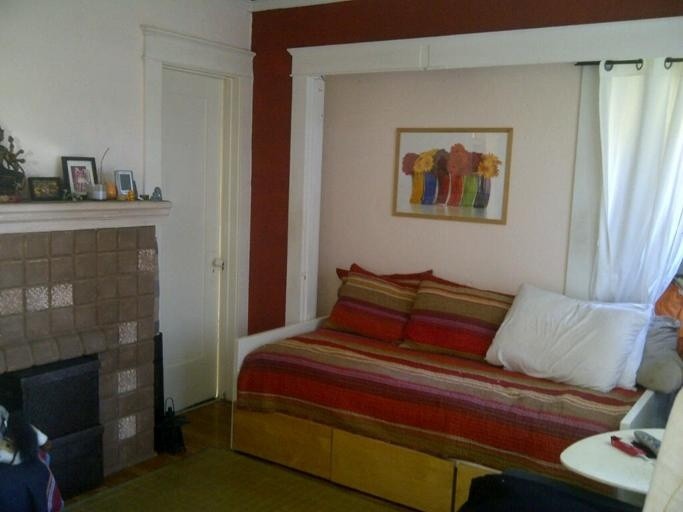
[
  {"left": 390, "top": 126, "right": 514, "bottom": 226},
  {"left": 29, "top": 154, "right": 98, "bottom": 200}
]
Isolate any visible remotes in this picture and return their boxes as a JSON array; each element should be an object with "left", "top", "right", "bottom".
[{"left": 635, "top": 431, "right": 662, "bottom": 456}]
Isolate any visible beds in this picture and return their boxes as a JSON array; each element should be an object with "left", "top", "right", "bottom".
[{"left": 229, "top": 299, "right": 676, "bottom": 512}]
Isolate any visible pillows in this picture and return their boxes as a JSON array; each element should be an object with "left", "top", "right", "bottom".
[
  {"left": 399, "top": 271, "right": 516, "bottom": 364},
  {"left": 322, "top": 264, "right": 433, "bottom": 345},
  {"left": 636, "top": 316, "right": 683, "bottom": 395},
  {"left": 484, "top": 281, "right": 654, "bottom": 394}
]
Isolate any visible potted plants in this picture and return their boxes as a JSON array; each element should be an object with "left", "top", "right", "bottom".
[{"left": 0, "top": 127, "right": 27, "bottom": 203}]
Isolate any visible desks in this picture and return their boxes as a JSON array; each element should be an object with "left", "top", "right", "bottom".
[{"left": 559, "top": 428, "right": 668, "bottom": 495}]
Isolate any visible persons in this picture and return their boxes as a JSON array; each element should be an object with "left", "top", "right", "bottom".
[{"left": 76, "top": 168, "right": 89, "bottom": 192}]
